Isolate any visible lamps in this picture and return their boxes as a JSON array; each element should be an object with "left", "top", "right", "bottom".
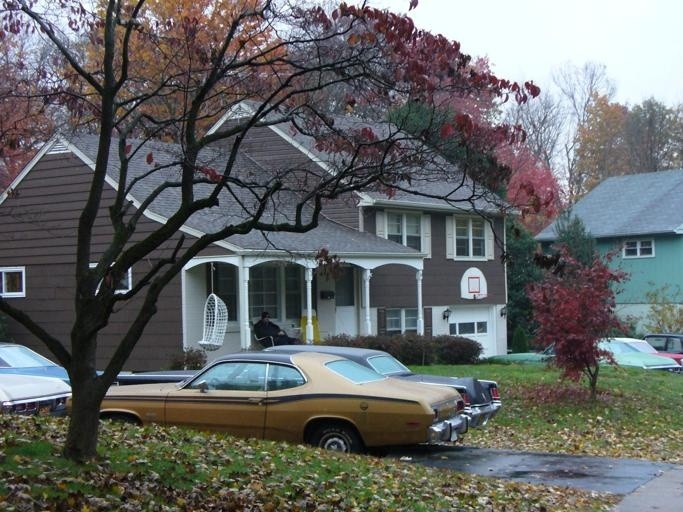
[
  {"left": 500, "top": 306, "right": 507, "bottom": 318},
  {"left": 442, "top": 307, "right": 451, "bottom": 322}
]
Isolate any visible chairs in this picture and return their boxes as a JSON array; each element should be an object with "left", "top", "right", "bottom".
[{"left": 196, "top": 294, "right": 227, "bottom": 352}]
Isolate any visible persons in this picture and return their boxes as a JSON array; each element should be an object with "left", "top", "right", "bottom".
[{"left": 253, "top": 311, "right": 291, "bottom": 348}]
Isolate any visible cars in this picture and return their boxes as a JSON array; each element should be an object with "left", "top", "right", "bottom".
[
  {"left": 504, "top": 331, "right": 682, "bottom": 374},
  {"left": 114, "top": 344, "right": 502, "bottom": 429},
  {"left": 0, "top": 343, "right": 72, "bottom": 417},
  {"left": 65, "top": 350, "right": 473, "bottom": 457}
]
